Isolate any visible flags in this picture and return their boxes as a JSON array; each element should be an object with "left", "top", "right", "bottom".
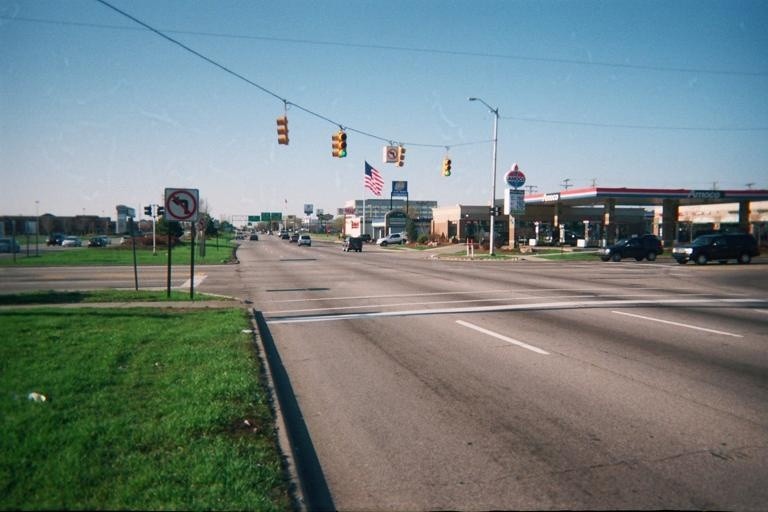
[{"left": 365, "top": 160, "right": 385, "bottom": 198}]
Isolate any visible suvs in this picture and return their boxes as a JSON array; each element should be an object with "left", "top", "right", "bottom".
[
  {"left": 297, "top": 235, "right": 313, "bottom": 246},
  {"left": 480, "top": 231, "right": 508, "bottom": 245},
  {"left": 45, "top": 232, "right": 64, "bottom": 246}
]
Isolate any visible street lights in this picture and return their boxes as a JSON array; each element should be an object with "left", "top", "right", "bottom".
[
  {"left": 284, "top": 199, "right": 288, "bottom": 231},
  {"left": 34, "top": 200, "right": 41, "bottom": 256},
  {"left": 468, "top": 97, "right": 500, "bottom": 255}
]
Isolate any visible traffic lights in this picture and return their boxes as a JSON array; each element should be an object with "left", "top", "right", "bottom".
[
  {"left": 397, "top": 146, "right": 406, "bottom": 168},
  {"left": 332, "top": 133, "right": 339, "bottom": 157},
  {"left": 339, "top": 131, "right": 348, "bottom": 158},
  {"left": 144, "top": 205, "right": 152, "bottom": 218},
  {"left": 157, "top": 205, "right": 164, "bottom": 216},
  {"left": 276, "top": 115, "right": 290, "bottom": 145},
  {"left": 443, "top": 159, "right": 451, "bottom": 177}
]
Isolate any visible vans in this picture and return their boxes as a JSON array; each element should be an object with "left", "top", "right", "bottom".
[
  {"left": 543, "top": 228, "right": 765, "bottom": 266},
  {"left": 342, "top": 236, "right": 363, "bottom": 252}
]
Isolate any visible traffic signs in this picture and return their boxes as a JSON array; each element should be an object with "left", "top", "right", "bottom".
[
  {"left": 248, "top": 216, "right": 260, "bottom": 222},
  {"left": 260, "top": 212, "right": 271, "bottom": 222},
  {"left": 270, "top": 212, "right": 283, "bottom": 222}
]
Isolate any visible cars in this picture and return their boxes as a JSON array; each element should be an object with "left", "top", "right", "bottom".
[
  {"left": 235, "top": 227, "right": 307, "bottom": 243},
  {"left": 87, "top": 234, "right": 131, "bottom": 248},
  {"left": 0, "top": 238, "right": 20, "bottom": 253},
  {"left": 61, "top": 235, "right": 82, "bottom": 247},
  {"left": 375, "top": 232, "right": 407, "bottom": 246},
  {"left": 357, "top": 234, "right": 371, "bottom": 241}
]
